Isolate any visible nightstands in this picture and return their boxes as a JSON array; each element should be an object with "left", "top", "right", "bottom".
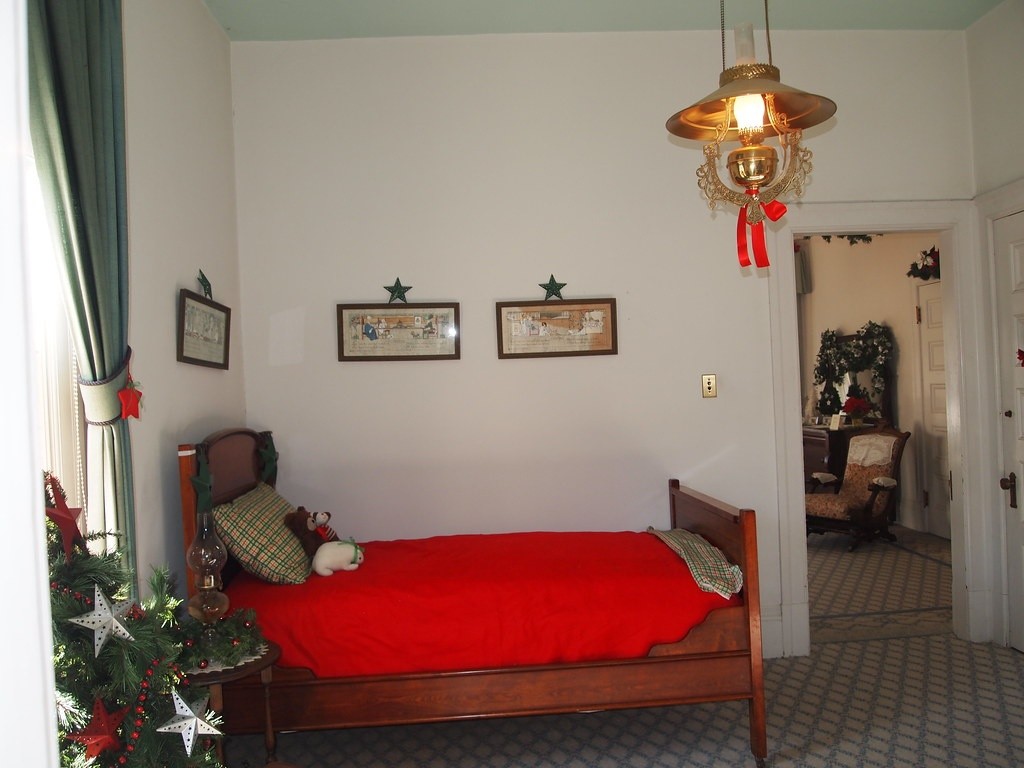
[{"left": 183, "top": 637, "right": 281, "bottom": 768}]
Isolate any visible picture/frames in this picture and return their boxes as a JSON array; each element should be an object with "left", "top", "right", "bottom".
[
  {"left": 337, "top": 302, "right": 461, "bottom": 362},
  {"left": 495, "top": 298, "right": 618, "bottom": 360},
  {"left": 176, "top": 288, "right": 231, "bottom": 371}
]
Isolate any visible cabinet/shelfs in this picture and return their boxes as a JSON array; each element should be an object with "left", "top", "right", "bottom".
[{"left": 803, "top": 423, "right": 877, "bottom": 495}]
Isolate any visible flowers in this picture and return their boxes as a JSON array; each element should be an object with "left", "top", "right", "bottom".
[{"left": 906, "top": 244, "right": 940, "bottom": 280}]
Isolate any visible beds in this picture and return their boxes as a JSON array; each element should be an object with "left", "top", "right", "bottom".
[{"left": 177, "top": 427, "right": 768, "bottom": 768}]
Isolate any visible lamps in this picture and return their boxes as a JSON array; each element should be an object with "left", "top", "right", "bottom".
[
  {"left": 186, "top": 512, "right": 230, "bottom": 634},
  {"left": 665, "top": 0, "right": 838, "bottom": 267}
]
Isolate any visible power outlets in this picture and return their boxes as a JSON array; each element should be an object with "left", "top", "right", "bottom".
[{"left": 701, "top": 374, "right": 717, "bottom": 398}]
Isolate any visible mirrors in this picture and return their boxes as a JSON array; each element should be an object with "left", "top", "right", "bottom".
[{"left": 827, "top": 334, "right": 892, "bottom": 424}]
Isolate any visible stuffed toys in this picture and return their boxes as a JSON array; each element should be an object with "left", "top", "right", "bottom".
[
  {"left": 284, "top": 506, "right": 324, "bottom": 559},
  {"left": 311, "top": 537, "right": 364, "bottom": 577},
  {"left": 308, "top": 511, "right": 340, "bottom": 541}
]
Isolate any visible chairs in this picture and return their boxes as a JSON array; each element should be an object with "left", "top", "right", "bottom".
[{"left": 806, "top": 418, "right": 911, "bottom": 551}]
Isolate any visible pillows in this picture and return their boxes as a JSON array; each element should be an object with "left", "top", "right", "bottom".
[{"left": 212, "top": 480, "right": 313, "bottom": 585}]
